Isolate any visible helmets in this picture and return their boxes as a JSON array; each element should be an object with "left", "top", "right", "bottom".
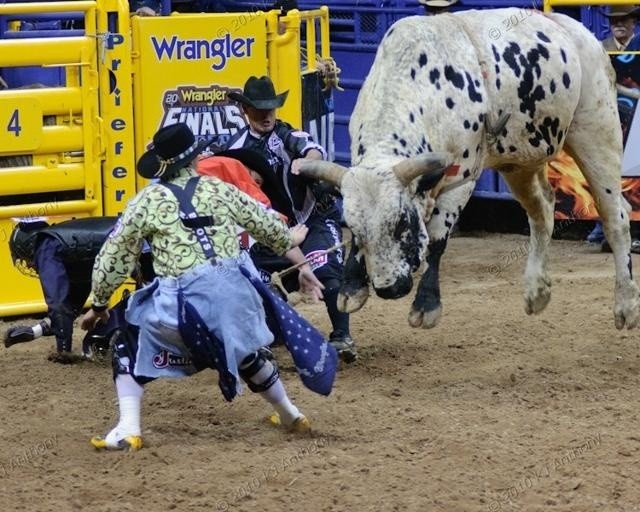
[{"left": 10, "top": 219, "right": 51, "bottom": 278}]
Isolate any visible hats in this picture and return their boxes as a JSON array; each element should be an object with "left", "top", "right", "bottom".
[
  {"left": 597, "top": 4, "right": 640, "bottom": 18},
  {"left": 136, "top": 122, "right": 219, "bottom": 179},
  {"left": 227, "top": 75, "right": 291, "bottom": 111},
  {"left": 418, "top": 0, "right": 458, "bottom": 7}
]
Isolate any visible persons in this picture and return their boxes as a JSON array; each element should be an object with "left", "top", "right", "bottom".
[
  {"left": 598, "top": 4, "right": 640, "bottom": 52},
  {"left": 81, "top": 122, "right": 329, "bottom": 453},
  {"left": 3, "top": 214, "right": 156, "bottom": 363},
  {"left": 194, "top": 148, "right": 326, "bottom": 303},
  {"left": 200, "top": 76, "right": 357, "bottom": 365}
]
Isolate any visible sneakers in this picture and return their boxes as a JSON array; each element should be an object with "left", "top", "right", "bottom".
[
  {"left": 327, "top": 330, "right": 361, "bottom": 364},
  {"left": 90, "top": 434, "right": 143, "bottom": 452},
  {"left": 270, "top": 414, "right": 312, "bottom": 434}
]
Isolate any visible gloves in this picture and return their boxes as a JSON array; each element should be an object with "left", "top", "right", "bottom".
[{"left": 3, "top": 325, "right": 36, "bottom": 348}]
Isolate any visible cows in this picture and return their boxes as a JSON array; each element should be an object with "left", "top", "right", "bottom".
[{"left": 290, "top": 6, "right": 640, "bottom": 330}]
